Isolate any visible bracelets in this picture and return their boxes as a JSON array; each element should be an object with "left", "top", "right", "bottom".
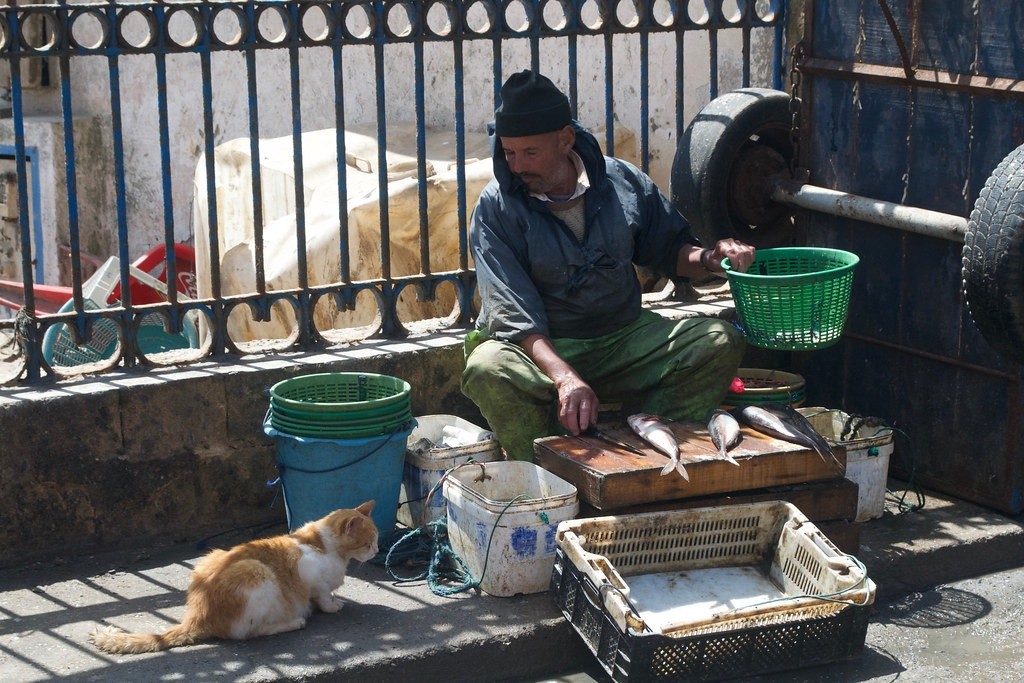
[{"left": 698, "top": 247, "right": 716, "bottom": 272}]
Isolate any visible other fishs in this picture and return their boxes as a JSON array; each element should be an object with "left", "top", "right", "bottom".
[
  {"left": 626, "top": 413, "right": 689, "bottom": 482},
  {"left": 735, "top": 404, "right": 826, "bottom": 464},
  {"left": 757, "top": 402, "right": 846, "bottom": 469},
  {"left": 706, "top": 408, "right": 741, "bottom": 466}
]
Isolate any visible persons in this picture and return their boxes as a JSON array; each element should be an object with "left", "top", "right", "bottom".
[{"left": 459, "top": 68, "right": 757, "bottom": 463}]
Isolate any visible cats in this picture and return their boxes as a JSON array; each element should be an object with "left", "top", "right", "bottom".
[{"left": 86, "top": 500, "right": 379, "bottom": 656}]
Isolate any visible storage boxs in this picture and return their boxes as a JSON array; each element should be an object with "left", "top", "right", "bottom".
[
  {"left": 547, "top": 500, "right": 874, "bottom": 683},
  {"left": 395, "top": 414, "right": 498, "bottom": 533},
  {"left": 445, "top": 460, "right": 579, "bottom": 597},
  {"left": 793, "top": 406, "right": 925, "bottom": 522}
]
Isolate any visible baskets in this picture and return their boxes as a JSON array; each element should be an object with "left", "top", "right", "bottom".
[
  {"left": 42, "top": 296, "right": 199, "bottom": 368},
  {"left": 270, "top": 372, "right": 412, "bottom": 438},
  {"left": 548, "top": 499, "right": 878, "bottom": 683},
  {"left": 721, "top": 246, "right": 858, "bottom": 351},
  {"left": 720, "top": 369, "right": 804, "bottom": 413}
]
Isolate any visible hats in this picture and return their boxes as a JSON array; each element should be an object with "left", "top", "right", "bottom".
[{"left": 495, "top": 69, "right": 573, "bottom": 137}]
